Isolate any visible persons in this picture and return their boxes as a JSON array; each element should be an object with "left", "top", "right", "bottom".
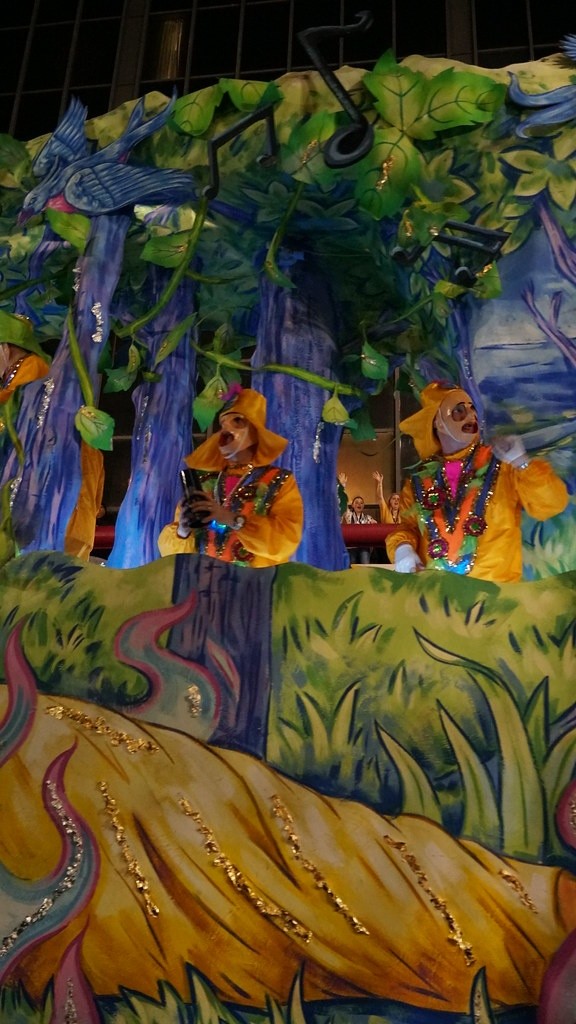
[
  {"left": 336, "top": 472, "right": 377, "bottom": 524},
  {"left": 157, "top": 387, "right": 304, "bottom": 569},
  {"left": 0, "top": 309, "right": 105, "bottom": 566},
  {"left": 384, "top": 379, "right": 568, "bottom": 585},
  {"left": 371, "top": 470, "right": 402, "bottom": 524}
]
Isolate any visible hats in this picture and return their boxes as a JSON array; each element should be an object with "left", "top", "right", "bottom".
[
  {"left": 398, "top": 381, "right": 463, "bottom": 461},
  {"left": 184, "top": 385, "right": 288, "bottom": 472}
]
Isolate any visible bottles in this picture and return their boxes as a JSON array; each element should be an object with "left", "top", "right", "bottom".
[{"left": 179, "top": 468, "right": 213, "bottom": 528}]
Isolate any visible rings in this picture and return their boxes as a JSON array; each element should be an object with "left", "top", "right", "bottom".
[{"left": 208, "top": 504, "right": 212, "bottom": 509}]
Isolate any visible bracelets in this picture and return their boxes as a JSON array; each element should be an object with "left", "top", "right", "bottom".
[{"left": 517, "top": 461, "right": 529, "bottom": 470}]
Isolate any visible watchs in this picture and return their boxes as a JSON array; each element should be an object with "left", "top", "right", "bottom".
[{"left": 233, "top": 515, "right": 245, "bottom": 531}]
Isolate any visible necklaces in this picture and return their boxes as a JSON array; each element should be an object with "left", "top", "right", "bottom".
[
  {"left": 390, "top": 507, "right": 399, "bottom": 523},
  {"left": 435, "top": 445, "right": 477, "bottom": 532},
  {"left": 410, "top": 454, "right": 502, "bottom": 575},
  {"left": 214, "top": 467, "right": 250, "bottom": 507}
]
